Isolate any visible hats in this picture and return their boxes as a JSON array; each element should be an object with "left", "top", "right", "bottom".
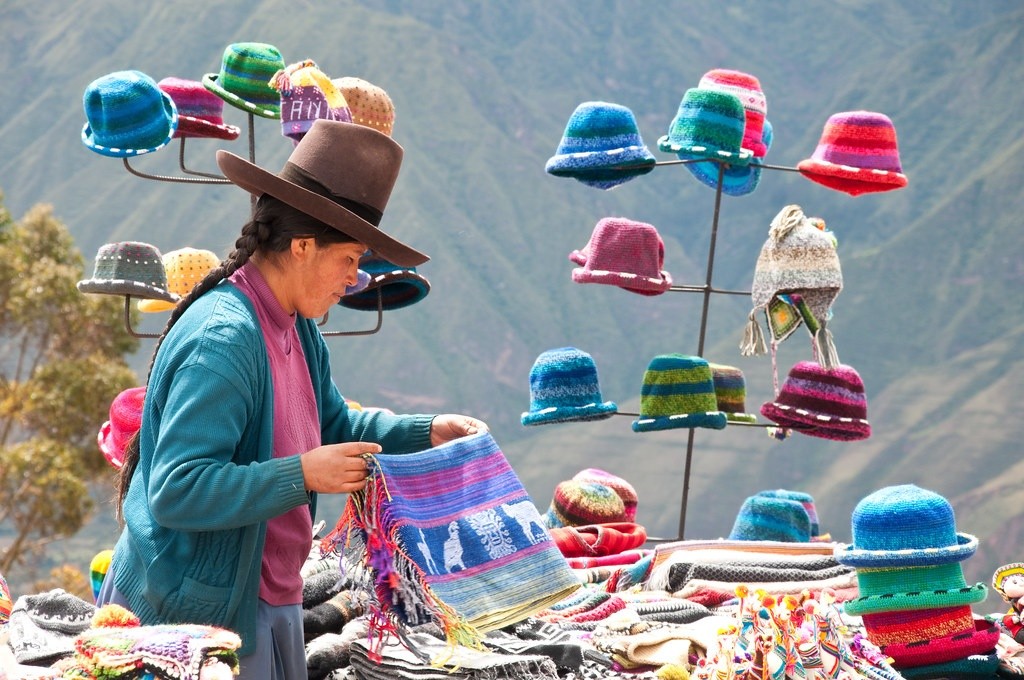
[
  {"left": 97, "top": 387, "right": 146, "bottom": 471},
  {"left": 569, "top": 216, "right": 672, "bottom": 297},
  {"left": 532, "top": 466, "right": 1001, "bottom": 680},
  {"left": 0, "top": 571, "right": 243, "bottom": 680},
  {"left": 76, "top": 241, "right": 221, "bottom": 312},
  {"left": 520, "top": 346, "right": 618, "bottom": 427},
  {"left": 216, "top": 119, "right": 431, "bottom": 268},
  {"left": 631, "top": 353, "right": 872, "bottom": 443},
  {"left": 796, "top": 111, "right": 909, "bottom": 197},
  {"left": 82, "top": 42, "right": 395, "bottom": 157},
  {"left": 657, "top": 68, "right": 774, "bottom": 197},
  {"left": 545, "top": 101, "right": 656, "bottom": 191},
  {"left": 337, "top": 247, "right": 430, "bottom": 313},
  {"left": 739, "top": 204, "right": 845, "bottom": 436}
]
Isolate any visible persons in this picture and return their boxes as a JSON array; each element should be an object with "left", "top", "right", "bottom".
[{"left": 94, "top": 118, "right": 489, "bottom": 680}]
